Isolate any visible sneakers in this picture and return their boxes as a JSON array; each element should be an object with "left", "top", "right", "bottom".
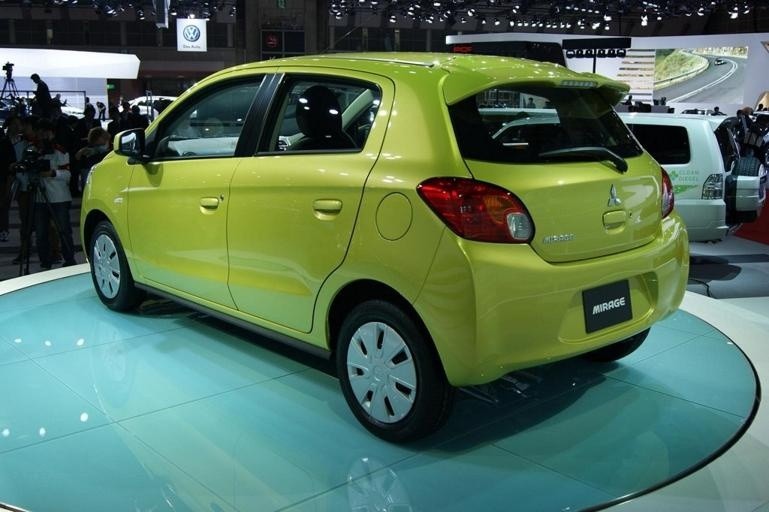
[
  {"left": 0, "top": 230, "right": 9, "bottom": 241},
  {"left": 13, "top": 256, "right": 28, "bottom": 263}
]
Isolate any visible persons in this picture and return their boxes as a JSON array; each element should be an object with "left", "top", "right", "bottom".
[
  {"left": 1, "top": 72, "right": 172, "bottom": 278},
  {"left": 480, "top": 94, "right": 769, "bottom": 168}
]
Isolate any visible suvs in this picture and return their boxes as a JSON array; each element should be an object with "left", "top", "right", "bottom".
[
  {"left": 75, "top": 47, "right": 695, "bottom": 447},
  {"left": 477, "top": 107, "right": 769, "bottom": 244},
  {"left": 0, "top": 89, "right": 182, "bottom": 132}
]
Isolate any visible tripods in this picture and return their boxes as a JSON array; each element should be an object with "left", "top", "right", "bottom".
[
  {"left": 1, "top": 78, "right": 20, "bottom": 99},
  {"left": 16, "top": 184, "right": 77, "bottom": 276}
]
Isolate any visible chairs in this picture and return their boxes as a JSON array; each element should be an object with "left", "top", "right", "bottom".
[{"left": 285, "top": 84, "right": 359, "bottom": 150}]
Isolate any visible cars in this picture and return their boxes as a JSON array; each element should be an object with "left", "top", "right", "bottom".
[
  {"left": 715, "top": 58, "right": 725, "bottom": 66},
  {"left": 84, "top": 297, "right": 726, "bottom": 512}
]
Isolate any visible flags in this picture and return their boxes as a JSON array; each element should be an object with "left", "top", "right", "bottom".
[{"left": 176, "top": 18, "right": 209, "bottom": 53}]
